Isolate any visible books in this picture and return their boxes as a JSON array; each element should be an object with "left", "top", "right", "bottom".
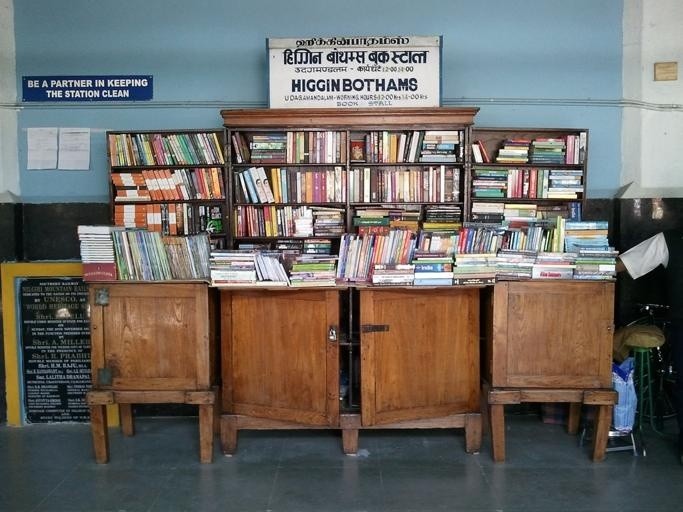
[
  {"left": 230, "top": 131, "right": 347, "bottom": 164},
  {"left": 470, "top": 166, "right": 584, "bottom": 199},
  {"left": 350, "top": 130, "right": 465, "bottom": 163},
  {"left": 111, "top": 167, "right": 226, "bottom": 202},
  {"left": 210, "top": 238, "right": 339, "bottom": 288},
  {"left": 114, "top": 203, "right": 222, "bottom": 235},
  {"left": 349, "top": 165, "right": 460, "bottom": 203},
  {"left": 470, "top": 202, "right": 582, "bottom": 231},
  {"left": 351, "top": 204, "right": 462, "bottom": 235},
  {"left": 336, "top": 215, "right": 619, "bottom": 288},
  {"left": 232, "top": 205, "right": 346, "bottom": 237},
  {"left": 472, "top": 131, "right": 586, "bottom": 165},
  {"left": 75, "top": 224, "right": 209, "bottom": 283},
  {"left": 233, "top": 166, "right": 347, "bottom": 204},
  {"left": 108, "top": 133, "right": 225, "bottom": 167}
]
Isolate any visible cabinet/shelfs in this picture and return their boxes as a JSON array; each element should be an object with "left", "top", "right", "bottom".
[
  {"left": 468, "top": 126, "right": 589, "bottom": 234},
  {"left": 487, "top": 279, "right": 618, "bottom": 462},
  {"left": 219, "top": 285, "right": 487, "bottom": 453},
  {"left": 106, "top": 129, "right": 229, "bottom": 250},
  {"left": 84, "top": 281, "right": 219, "bottom": 464},
  {"left": 220, "top": 108, "right": 480, "bottom": 247}
]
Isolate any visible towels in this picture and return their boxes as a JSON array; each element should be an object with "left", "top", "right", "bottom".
[{"left": 619, "top": 233, "right": 670, "bottom": 280}]
